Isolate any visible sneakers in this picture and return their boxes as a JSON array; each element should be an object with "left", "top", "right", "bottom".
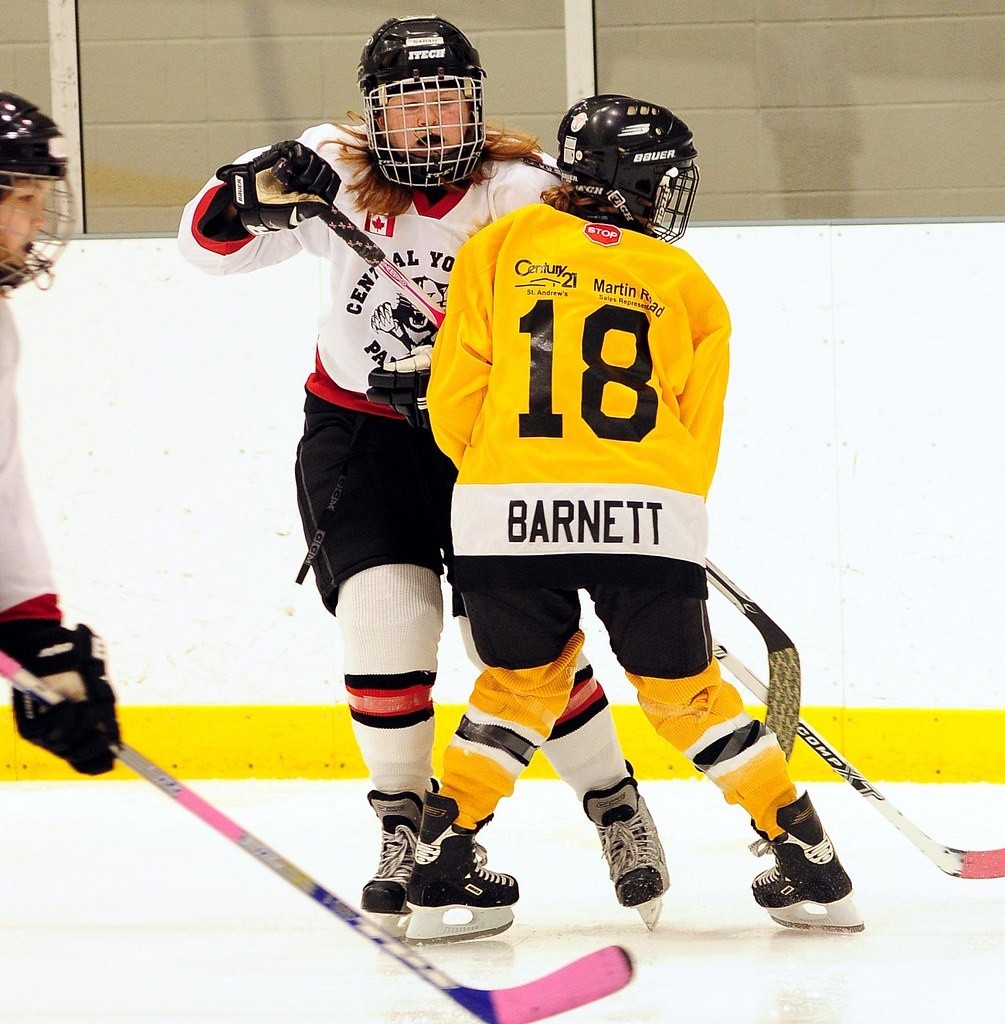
[
  {"left": 751, "top": 786, "right": 865, "bottom": 932},
  {"left": 361, "top": 778, "right": 440, "bottom": 940},
  {"left": 404, "top": 790, "right": 520, "bottom": 947},
  {"left": 582, "top": 757, "right": 671, "bottom": 931}
]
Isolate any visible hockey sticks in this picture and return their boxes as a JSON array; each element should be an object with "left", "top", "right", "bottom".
[
  {"left": 270, "top": 155, "right": 1005, "bottom": 880},
  {"left": 705, "top": 557, "right": 801, "bottom": 761},
  {"left": 1, "top": 651, "right": 633, "bottom": 1024}
]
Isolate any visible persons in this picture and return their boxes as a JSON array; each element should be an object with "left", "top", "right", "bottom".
[
  {"left": 177, "top": 16, "right": 671, "bottom": 935},
  {"left": 0, "top": 90, "right": 118, "bottom": 775},
  {"left": 404, "top": 94, "right": 864, "bottom": 945}
]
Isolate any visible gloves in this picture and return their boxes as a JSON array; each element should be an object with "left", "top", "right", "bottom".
[{"left": 0, "top": 618, "right": 122, "bottom": 776}]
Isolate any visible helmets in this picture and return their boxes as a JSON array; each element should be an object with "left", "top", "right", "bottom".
[
  {"left": 357, "top": 15, "right": 485, "bottom": 185},
  {"left": 0, "top": 90, "right": 77, "bottom": 291},
  {"left": 557, "top": 94, "right": 700, "bottom": 244}
]
